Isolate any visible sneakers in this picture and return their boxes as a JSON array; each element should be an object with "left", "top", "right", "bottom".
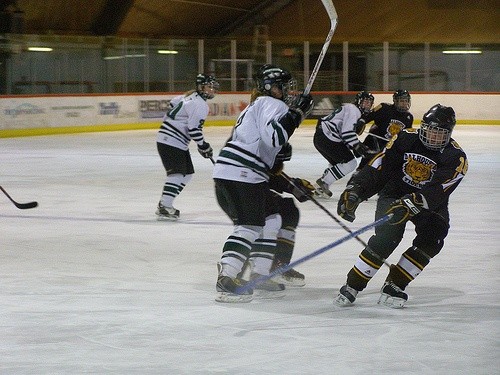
[
  {"left": 237, "top": 271, "right": 287, "bottom": 298},
  {"left": 155, "top": 201, "right": 180, "bottom": 221},
  {"left": 216, "top": 262, "right": 253, "bottom": 302},
  {"left": 335, "top": 284, "right": 359, "bottom": 307},
  {"left": 268, "top": 257, "right": 306, "bottom": 287},
  {"left": 376, "top": 281, "right": 408, "bottom": 309},
  {"left": 316, "top": 168, "right": 332, "bottom": 199}
]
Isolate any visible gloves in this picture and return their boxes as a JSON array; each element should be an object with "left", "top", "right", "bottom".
[
  {"left": 337, "top": 188, "right": 363, "bottom": 222},
  {"left": 354, "top": 120, "right": 366, "bottom": 136},
  {"left": 288, "top": 91, "right": 315, "bottom": 120},
  {"left": 276, "top": 143, "right": 292, "bottom": 161},
  {"left": 197, "top": 142, "right": 213, "bottom": 158},
  {"left": 353, "top": 142, "right": 369, "bottom": 158},
  {"left": 284, "top": 177, "right": 315, "bottom": 202},
  {"left": 385, "top": 193, "right": 426, "bottom": 226}
]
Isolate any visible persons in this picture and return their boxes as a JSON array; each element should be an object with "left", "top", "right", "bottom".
[
  {"left": 154, "top": 72, "right": 220, "bottom": 221},
  {"left": 213, "top": 65, "right": 315, "bottom": 302},
  {"left": 313, "top": 90, "right": 374, "bottom": 197},
  {"left": 347, "top": 90, "right": 414, "bottom": 188},
  {"left": 337, "top": 104, "right": 468, "bottom": 308}
]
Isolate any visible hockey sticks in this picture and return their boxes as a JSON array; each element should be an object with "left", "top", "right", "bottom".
[
  {"left": 367, "top": 137, "right": 380, "bottom": 155},
  {"left": 302, "top": 0, "right": 339, "bottom": 97},
  {"left": 209, "top": 155, "right": 216, "bottom": 167},
  {"left": 0, "top": 186, "right": 38, "bottom": 210},
  {"left": 363, "top": 130, "right": 389, "bottom": 142},
  {"left": 218, "top": 213, "right": 394, "bottom": 295},
  {"left": 310, "top": 197, "right": 392, "bottom": 267}
]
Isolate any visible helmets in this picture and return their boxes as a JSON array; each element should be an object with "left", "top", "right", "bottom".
[
  {"left": 195, "top": 73, "right": 216, "bottom": 99},
  {"left": 420, "top": 104, "right": 456, "bottom": 151},
  {"left": 256, "top": 63, "right": 292, "bottom": 99},
  {"left": 393, "top": 89, "right": 411, "bottom": 111},
  {"left": 355, "top": 91, "right": 374, "bottom": 114}
]
